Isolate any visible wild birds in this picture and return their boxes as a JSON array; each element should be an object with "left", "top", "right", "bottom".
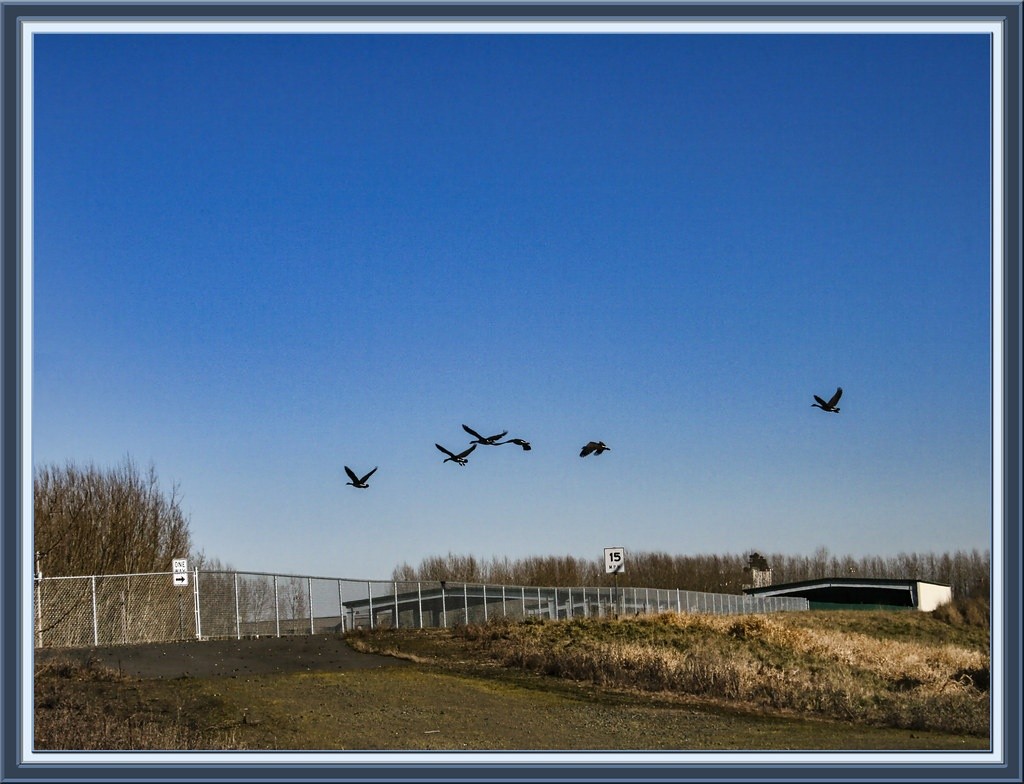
[
  {"left": 462, "top": 424, "right": 508, "bottom": 445},
  {"left": 579, "top": 441, "right": 611, "bottom": 458},
  {"left": 810, "top": 387, "right": 843, "bottom": 414},
  {"left": 493, "top": 439, "right": 531, "bottom": 450},
  {"left": 435, "top": 443, "right": 478, "bottom": 466}
]
